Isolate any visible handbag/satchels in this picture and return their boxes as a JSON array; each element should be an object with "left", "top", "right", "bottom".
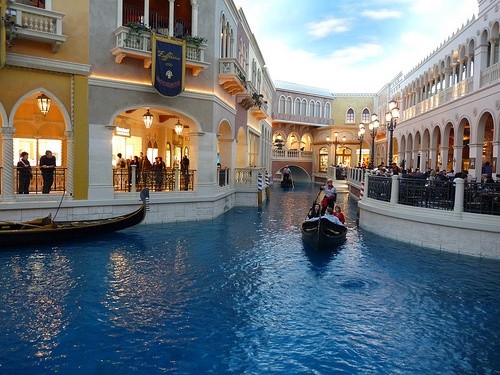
[{"left": 27, "top": 170, "right": 32, "bottom": 178}]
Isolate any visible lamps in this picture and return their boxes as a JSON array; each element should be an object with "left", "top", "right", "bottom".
[
  {"left": 143, "top": 108, "right": 154, "bottom": 129},
  {"left": 36, "top": 93, "right": 52, "bottom": 116},
  {"left": 173, "top": 118, "right": 184, "bottom": 136}
]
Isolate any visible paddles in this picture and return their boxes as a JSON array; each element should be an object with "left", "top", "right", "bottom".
[
  {"left": 305, "top": 189, "right": 322, "bottom": 219},
  {"left": 290, "top": 174, "right": 294, "bottom": 188}
]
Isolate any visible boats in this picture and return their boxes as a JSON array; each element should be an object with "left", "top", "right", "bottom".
[
  {"left": 280, "top": 179, "right": 294, "bottom": 188},
  {"left": 0, "top": 186, "right": 149, "bottom": 244},
  {"left": 300, "top": 214, "right": 347, "bottom": 251}
]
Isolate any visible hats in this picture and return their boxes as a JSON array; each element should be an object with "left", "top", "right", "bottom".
[{"left": 327, "top": 179, "right": 333, "bottom": 184}]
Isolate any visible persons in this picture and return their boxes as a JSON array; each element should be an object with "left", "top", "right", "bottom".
[
  {"left": 325, "top": 207, "right": 332, "bottom": 216},
  {"left": 336, "top": 165, "right": 342, "bottom": 175},
  {"left": 284, "top": 165, "right": 291, "bottom": 181},
  {"left": 376, "top": 162, "right": 400, "bottom": 177},
  {"left": 460, "top": 170, "right": 472, "bottom": 182},
  {"left": 334, "top": 205, "right": 345, "bottom": 224},
  {"left": 402, "top": 168, "right": 412, "bottom": 178},
  {"left": 311, "top": 204, "right": 320, "bottom": 218},
  {"left": 182, "top": 156, "right": 189, "bottom": 173},
  {"left": 115, "top": 152, "right": 167, "bottom": 192},
  {"left": 17, "top": 152, "right": 33, "bottom": 194},
  {"left": 414, "top": 168, "right": 455, "bottom": 187},
  {"left": 482, "top": 162, "right": 494, "bottom": 183},
  {"left": 320, "top": 179, "right": 338, "bottom": 216},
  {"left": 41, "top": 150, "right": 56, "bottom": 195}
]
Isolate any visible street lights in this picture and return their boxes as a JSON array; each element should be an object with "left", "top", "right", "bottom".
[
  {"left": 385, "top": 100, "right": 399, "bottom": 169},
  {"left": 327, "top": 131, "right": 347, "bottom": 166},
  {"left": 358, "top": 122, "right": 365, "bottom": 168},
  {"left": 368, "top": 112, "right": 379, "bottom": 169}
]
